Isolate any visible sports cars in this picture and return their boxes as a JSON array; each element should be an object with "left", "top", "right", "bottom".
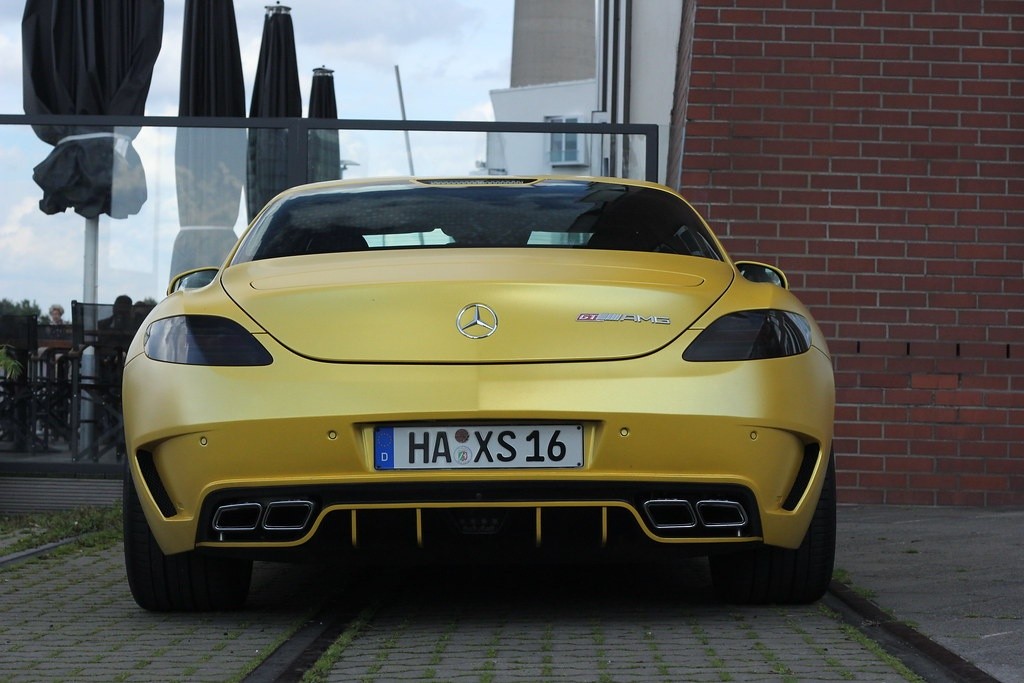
[{"left": 120, "top": 171, "right": 839, "bottom": 616}]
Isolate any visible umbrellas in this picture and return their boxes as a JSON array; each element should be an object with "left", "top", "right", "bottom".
[
  {"left": 22, "top": 0, "right": 164, "bottom": 462},
  {"left": 246, "top": 1, "right": 302, "bottom": 224},
  {"left": 307, "top": 66, "right": 341, "bottom": 184},
  {"left": 167, "top": 0, "right": 246, "bottom": 290}
]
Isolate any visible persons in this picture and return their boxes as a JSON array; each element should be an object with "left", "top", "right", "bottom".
[
  {"left": 97, "top": 295, "right": 157, "bottom": 330},
  {"left": 47, "top": 305, "right": 70, "bottom": 324}
]
